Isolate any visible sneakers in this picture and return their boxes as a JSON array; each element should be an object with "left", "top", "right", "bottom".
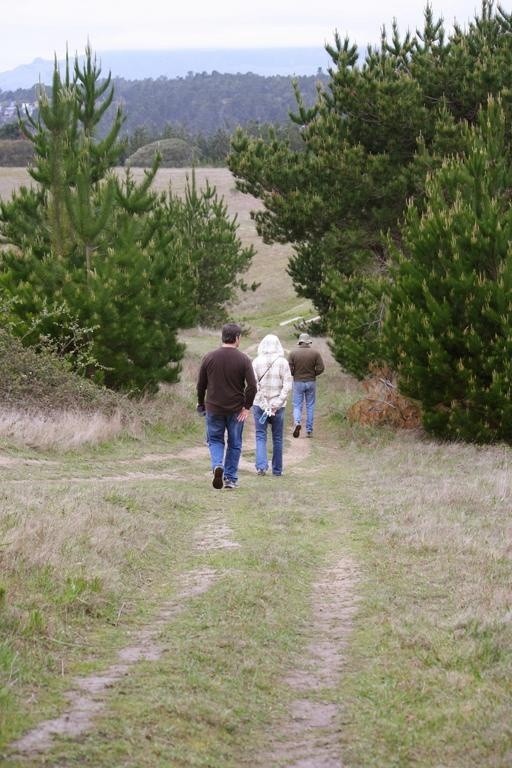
[
  {"left": 212, "top": 465, "right": 225, "bottom": 489},
  {"left": 293, "top": 424, "right": 301, "bottom": 438},
  {"left": 256, "top": 468, "right": 282, "bottom": 476},
  {"left": 224, "top": 479, "right": 240, "bottom": 489},
  {"left": 306, "top": 431, "right": 313, "bottom": 438}
]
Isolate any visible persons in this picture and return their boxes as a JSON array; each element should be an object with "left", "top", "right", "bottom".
[
  {"left": 288, "top": 333, "right": 325, "bottom": 437},
  {"left": 247, "top": 334, "right": 293, "bottom": 476},
  {"left": 196, "top": 323, "right": 257, "bottom": 489}
]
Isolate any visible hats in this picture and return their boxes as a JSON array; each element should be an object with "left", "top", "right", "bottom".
[{"left": 296, "top": 333, "right": 313, "bottom": 345}]
